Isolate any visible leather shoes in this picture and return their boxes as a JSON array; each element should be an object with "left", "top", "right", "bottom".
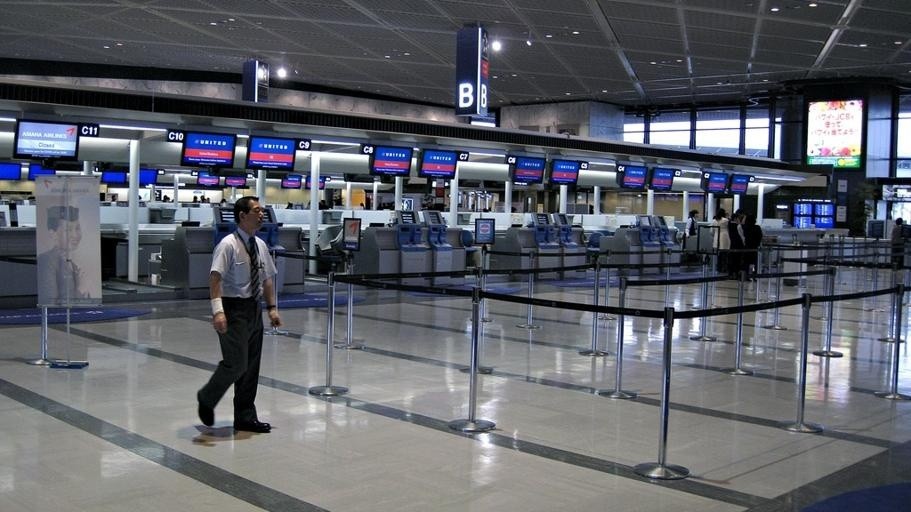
[
  {"left": 232, "top": 418, "right": 271, "bottom": 434},
  {"left": 195, "top": 388, "right": 215, "bottom": 427}
]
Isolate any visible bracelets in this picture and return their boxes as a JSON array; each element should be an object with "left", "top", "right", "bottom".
[
  {"left": 210, "top": 298, "right": 224, "bottom": 316},
  {"left": 266, "top": 305, "right": 276, "bottom": 309}
]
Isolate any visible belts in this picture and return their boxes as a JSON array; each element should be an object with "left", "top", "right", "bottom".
[{"left": 221, "top": 296, "right": 257, "bottom": 304}]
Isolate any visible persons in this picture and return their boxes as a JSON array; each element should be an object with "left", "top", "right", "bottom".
[
  {"left": 891, "top": 218, "right": 905, "bottom": 270},
  {"left": 37, "top": 206, "right": 84, "bottom": 304},
  {"left": 682, "top": 208, "right": 762, "bottom": 282},
  {"left": 197, "top": 196, "right": 283, "bottom": 432},
  {"left": 286, "top": 199, "right": 336, "bottom": 209},
  {"left": 360, "top": 201, "right": 395, "bottom": 210},
  {"left": 111, "top": 195, "right": 227, "bottom": 206}
]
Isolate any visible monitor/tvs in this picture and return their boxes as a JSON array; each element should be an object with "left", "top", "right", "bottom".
[
  {"left": 369, "top": 146, "right": 414, "bottom": 178},
  {"left": 708, "top": 173, "right": 729, "bottom": 194},
  {"left": 29, "top": 163, "right": 57, "bottom": 182},
  {"left": 512, "top": 157, "right": 547, "bottom": 185},
  {"left": 729, "top": 175, "right": 750, "bottom": 195},
  {"left": 306, "top": 176, "right": 327, "bottom": 191},
  {"left": 13, "top": 119, "right": 81, "bottom": 170},
  {"left": 550, "top": 159, "right": 581, "bottom": 186},
  {"left": 1, "top": 162, "right": 23, "bottom": 182},
  {"left": 621, "top": 165, "right": 649, "bottom": 191},
  {"left": 282, "top": 175, "right": 303, "bottom": 190},
  {"left": 140, "top": 168, "right": 159, "bottom": 186},
  {"left": 181, "top": 131, "right": 238, "bottom": 170},
  {"left": 650, "top": 168, "right": 676, "bottom": 192},
  {"left": 246, "top": 136, "right": 298, "bottom": 173},
  {"left": 102, "top": 170, "right": 127, "bottom": 185},
  {"left": 225, "top": 174, "right": 248, "bottom": 189},
  {"left": 419, "top": 149, "right": 459, "bottom": 180},
  {"left": 198, "top": 171, "right": 221, "bottom": 187}
]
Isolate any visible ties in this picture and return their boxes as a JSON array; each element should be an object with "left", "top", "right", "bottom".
[{"left": 247, "top": 236, "right": 262, "bottom": 303}]
